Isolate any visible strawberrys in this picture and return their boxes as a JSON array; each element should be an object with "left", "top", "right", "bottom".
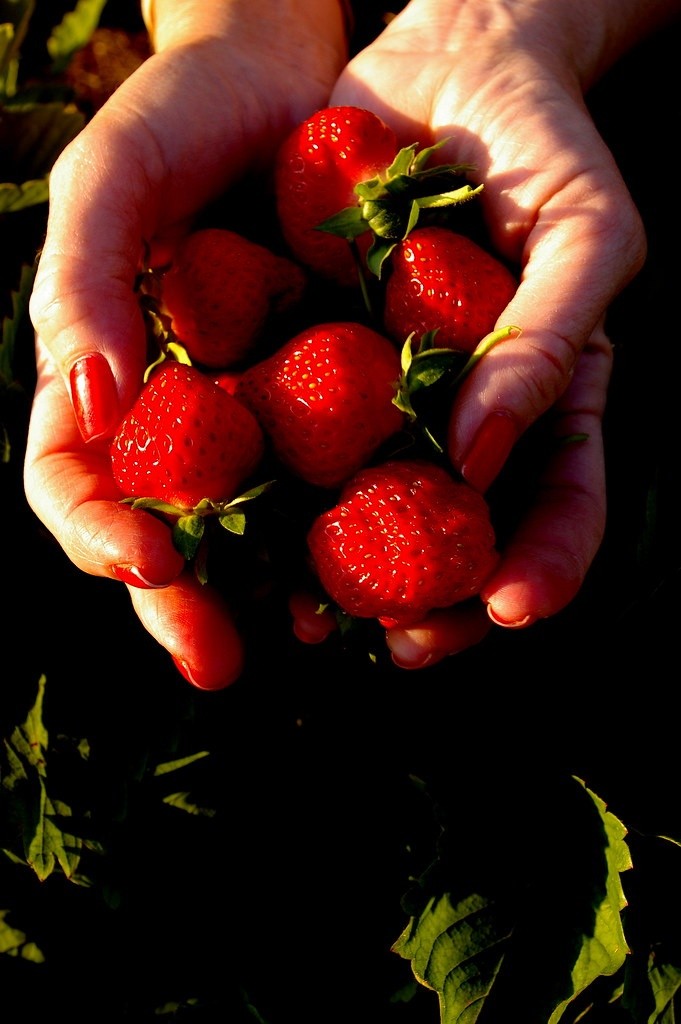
[{"left": 106, "top": 102, "right": 514, "bottom": 625}]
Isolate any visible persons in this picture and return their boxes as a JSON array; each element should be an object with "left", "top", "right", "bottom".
[{"left": 25, "top": 0, "right": 645, "bottom": 691}]
